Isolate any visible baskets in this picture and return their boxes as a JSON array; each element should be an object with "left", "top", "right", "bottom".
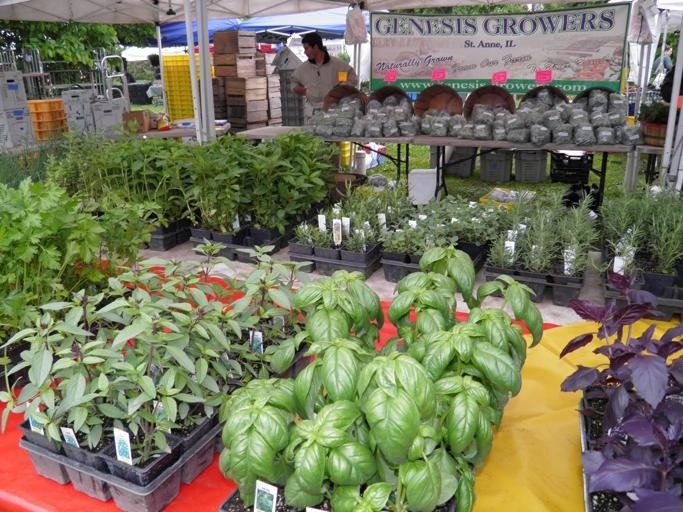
[
  {"left": 339, "top": 141, "right": 351, "bottom": 165},
  {"left": 162, "top": 54, "right": 202, "bottom": 120},
  {"left": 26, "top": 98, "right": 69, "bottom": 141},
  {"left": 639, "top": 122, "right": 667, "bottom": 147}
]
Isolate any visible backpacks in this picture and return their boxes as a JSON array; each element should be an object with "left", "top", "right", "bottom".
[{"left": 661, "top": 65, "right": 676, "bottom": 103}]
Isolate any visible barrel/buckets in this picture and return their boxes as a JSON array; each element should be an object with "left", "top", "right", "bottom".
[
  {"left": 354, "top": 149, "right": 366, "bottom": 172},
  {"left": 639, "top": 120, "right": 677, "bottom": 147},
  {"left": 367, "top": 87, "right": 413, "bottom": 115},
  {"left": 413, "top": 84, "right": 463, "bottom": 116},
  {"left": 324, "top": 84, "right": 367, "bottom": 115},
  {"left": 464, "top": 85, "right": 516, "bottom": 117},
  {"left": 520, "top": 85, "right": 569, "bottom": 105}
]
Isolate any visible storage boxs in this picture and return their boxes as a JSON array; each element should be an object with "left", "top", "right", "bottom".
[
  {"left": 123, "top": 111, "right": 150, "bottom": 133},
  {"left": 211, "top": 31, "right": 284, "bottom": 132}
]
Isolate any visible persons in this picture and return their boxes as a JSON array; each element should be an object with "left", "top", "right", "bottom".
[
  {"left": 291, "top": 32, "right": 358, "bottom": 118},
  {"left": 652, "top": 46, "right": 673, "bottom": 76}
]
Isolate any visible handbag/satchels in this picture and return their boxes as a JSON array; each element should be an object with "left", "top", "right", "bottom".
[{"left": 345, "top": 2, "right": 368, "bottom": 44}]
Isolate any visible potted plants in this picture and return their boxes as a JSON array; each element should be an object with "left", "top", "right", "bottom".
[
  {"left": 0, "top": 121, "right": 682, "bottom": 511},
  {"left": 638, "top": 101, "right": 669, "bottom": 146}
]
